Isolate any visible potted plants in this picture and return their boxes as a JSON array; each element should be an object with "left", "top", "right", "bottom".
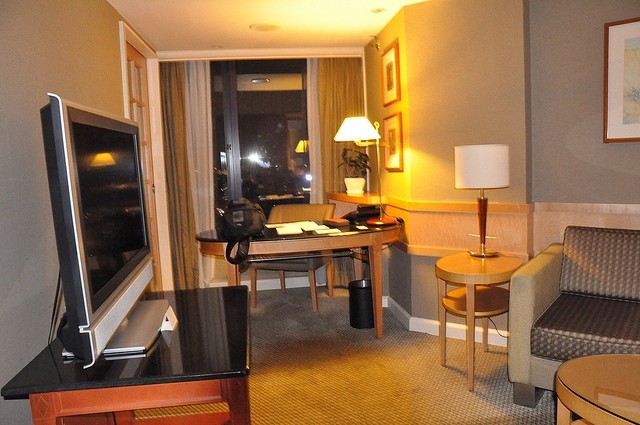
[{"left": 338, "top": 149, "right": 371, "bottom": 197}]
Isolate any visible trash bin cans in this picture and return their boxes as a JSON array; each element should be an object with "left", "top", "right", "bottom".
[{"left": 348, "top": 279, "right": 374, "bottom": 329}]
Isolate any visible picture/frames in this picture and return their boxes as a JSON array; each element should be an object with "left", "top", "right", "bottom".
[
  {"left": 383, "top": 112, "right": 404, "bottom": 172},
  {"left": 602, "top": 18, "right": 640, "bottom": 143},
  {"left": 380, "top": 37, "right": 401, "bottom": 107}
]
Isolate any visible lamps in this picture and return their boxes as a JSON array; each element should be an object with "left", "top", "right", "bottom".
[
  {"left": 295, "top": 140, "right": 308, "bottom": 154},
  {"left": 455, "top": 144, "right": 509, "bottom": 256},
  {"left": 333, "top": 116, "right": 383, "bottom": 148}
]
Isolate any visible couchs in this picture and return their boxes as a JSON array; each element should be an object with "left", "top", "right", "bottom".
[{"left": 507, "top": 225, "right": 640, "bottom": 407}]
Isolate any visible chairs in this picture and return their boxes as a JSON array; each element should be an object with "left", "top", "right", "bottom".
[{"left": 250, "top": 202, "right": 337, "bottom": 313}]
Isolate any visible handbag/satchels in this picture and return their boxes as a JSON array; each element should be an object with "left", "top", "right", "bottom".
[{"left": 221, "top": 198, "right": 267, "bottom": 265}]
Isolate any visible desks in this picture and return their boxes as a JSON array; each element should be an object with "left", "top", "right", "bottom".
[
  {"left": 195, "top": 214, "right": 401, "bottom": 338},
  {"left": 1, "top": 285, "right": 251, "bottom": 425}
]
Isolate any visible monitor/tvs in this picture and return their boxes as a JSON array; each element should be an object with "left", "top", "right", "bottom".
[{"left": 38, "top": 91, "right": 150, "bottom": 327}]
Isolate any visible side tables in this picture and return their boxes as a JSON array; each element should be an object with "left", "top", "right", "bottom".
[{"left": 434, "top": 253, "right": 523, "bottom": 391}]
